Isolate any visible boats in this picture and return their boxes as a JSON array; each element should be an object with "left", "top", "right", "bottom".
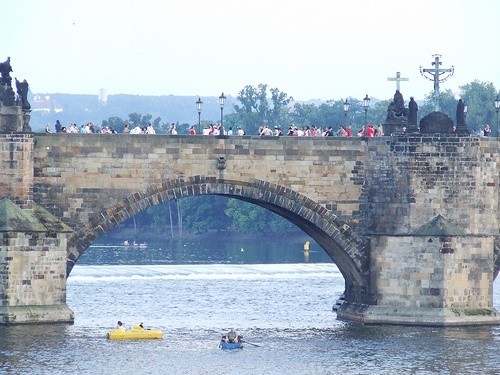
[
  {"left": 107, "top": 325, "right": 162, "bottom": 339},
  {"left": 220, "top": 336, "right": 243, "bottom": 349}
]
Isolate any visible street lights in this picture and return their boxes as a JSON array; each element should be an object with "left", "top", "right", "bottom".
[
  {"left": 196, "top": 97, "right": 203, "bottom": 133},
  {"left": 362, "top": 94, "right": 370, "bottom": 137},
  {"left": 343, "top": 98, "right": 350, "bottom": 125},
  {"left": 463, "top": 100, "right": 468, "bottom": 121},
  {"left": 494, "top": 95, "right": 499, "bottom": 135},
  {"left": 218, "top": 92, "right": 227, "bottom": 135}
]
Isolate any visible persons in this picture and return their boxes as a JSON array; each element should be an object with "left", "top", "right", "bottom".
[
  {"left": 228, "top": 126, "right": 233, "bottom": 136},
  {"left": 209, "top": 123, "right": 225, "bottom": 136},
  {"left": 358, "top": 124, "right": 384, "bottom": 136},
  {"left": 237, "top": 127, "right": 245, "bottom": 136},
  {"left": 258, "top": 125, "right": 283, "bottom": 137},
  {"left": 169, "top": 123, "right": 177, "bottom": 134},
  {"left": 124, "top": 123, "right": 156, "bottom": 135},
  {"left": 117, "top": 320, "right": 125, "bottom": 330},
  {"left": 484, "top": 125, "right": 491, "bottom": 136},
  {"left": 287, "top": 123, "right": 352, "bottom": 137},
  {"left": 139, "top": 322, "right": 145, "bottom": 328},
  {"left": 221, "top": 328, "right": 243, "bottom": 343},
  {"left": 44, "top": 120, "right": 117, "bottom": 134},
  {"left": 190, "top": 126, "right": 196, "bottom": 135}
]
